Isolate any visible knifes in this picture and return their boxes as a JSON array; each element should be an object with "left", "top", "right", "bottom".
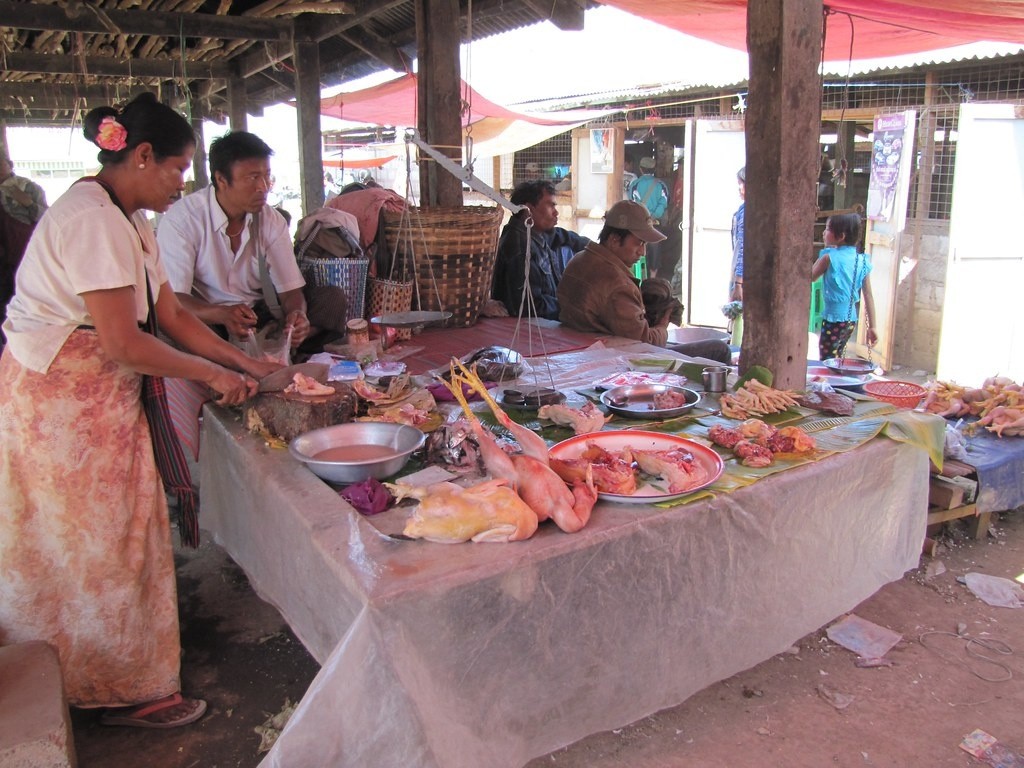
[{"left": 211, "top": 362, "right": 331, "bottom": 401}]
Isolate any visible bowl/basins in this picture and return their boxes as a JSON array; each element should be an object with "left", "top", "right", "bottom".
[
  {"left": 288, "top": 421, "right": 424, "bottom": 487},
  {"left": 807, "top": 365, "right": 873, "bottom": 392},
  {"left": 667, "top": 328, "right": 732, "bottom": 344}
]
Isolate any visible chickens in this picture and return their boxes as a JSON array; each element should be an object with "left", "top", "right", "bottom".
[
  {"left": 435, "top": 357, "right": 599, "bottom": 534},
  {"left": 383, "top": 478, "right": 538, "bottom": 544},
  {"left": 923, "top": 374, "right": 1024, "bottom": 439}
]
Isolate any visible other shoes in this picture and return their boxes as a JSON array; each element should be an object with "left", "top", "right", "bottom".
[{"left": 295, "top": 351, "right": 310, "bottom": 363}]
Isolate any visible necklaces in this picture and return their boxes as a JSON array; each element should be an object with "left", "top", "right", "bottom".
[{"left": 227, "top": 225, "right": 243, "bottom": 239}]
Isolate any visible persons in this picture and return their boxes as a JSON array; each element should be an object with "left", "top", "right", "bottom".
[
  {"left": 264, "top": 175, "right": 292, "bottom": 229},
  {"left": 621, "top": 154, "right": 637, "bottom": 202},
  {"left": 628, "top": 157, "right": 669, "bottom": 277},
  {"left": 0, "top": 141, "right": 49, "bottom": 344},
  {"left": 526, "top": 163, "right": 572, "bottom": 192},
  {"left": 491, "top": 181, "right": 597, "bottom": 321},
  {"left": 556, "top": 197, "right": 733, "bottom": 364},
  {"left": 155, "top": 128, "right": 312, "bottom": 463},
  {"left": 670, "top": 157, "right": 682, "bottom": 230},
  {"left": 812, "top": 210, "right": 879, "bottom": 359},
  {"left": 725, "top": 166, "right": 746, "bottom": 356},
  {"left": 0, "top": 91, "right": 284, "bottom": 731},
  {"left": 321, "top": 168, "right": 375, "bottom": 200}
]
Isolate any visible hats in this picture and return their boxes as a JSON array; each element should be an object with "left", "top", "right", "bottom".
[
  {"left": 604, "top": 200, "right": 668, "bottom": 244},
  {"left": 638, "top": 157, "right": 656, "bottom": 168},
  {"left": 739, "top": 167, "right": 746, "bottom": 181}
]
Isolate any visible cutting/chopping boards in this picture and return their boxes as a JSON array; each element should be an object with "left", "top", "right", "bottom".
[{"left": 243, "top": 379, "right": 357, "bottom": 442}]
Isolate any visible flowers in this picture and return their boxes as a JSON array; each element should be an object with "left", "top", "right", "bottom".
[{"left": 94, "top": 117, "right": 126, "bottom": 152}]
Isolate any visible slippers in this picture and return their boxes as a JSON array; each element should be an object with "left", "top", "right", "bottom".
[{"left": 101, "top": 692, "right": 209, "bottom": 729}]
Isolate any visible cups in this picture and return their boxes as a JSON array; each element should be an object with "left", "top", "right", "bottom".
[{"left": 702, "top": 365, "right": 728, "bottom": 392}]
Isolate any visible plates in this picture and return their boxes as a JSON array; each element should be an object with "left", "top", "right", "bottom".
[
  {"left": 599, "top": 384, "right": 700, "bottom": 419},
  {"left": 547, "top": 430, "right": 724, "bottom": 503}
]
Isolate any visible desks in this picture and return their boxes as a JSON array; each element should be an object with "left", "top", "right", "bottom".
[{"left": 158, "top": 316, "right": 1024, "bottom": 768}]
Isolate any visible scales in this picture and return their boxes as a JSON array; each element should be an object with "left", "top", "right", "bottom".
[{"left": 371, "top": 0, "right": 567, "bottom": 410}]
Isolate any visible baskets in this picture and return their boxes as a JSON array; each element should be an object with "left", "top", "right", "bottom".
[
  {"left": 367, "top": 278, "right": 413, "bottom": 341},
  {"left": 297, "top": 256, "right": 370, "bottom": 335},
  {"left": 863, "top": 380, "right": 927, "bottom": 409},
  {"left": 383, "top": 203, "right": 504, "bottom": 327}
]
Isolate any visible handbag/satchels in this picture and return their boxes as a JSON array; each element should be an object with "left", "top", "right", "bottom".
[{"left": 245, "top": 325, "right": 295, "bottom": 366}]
